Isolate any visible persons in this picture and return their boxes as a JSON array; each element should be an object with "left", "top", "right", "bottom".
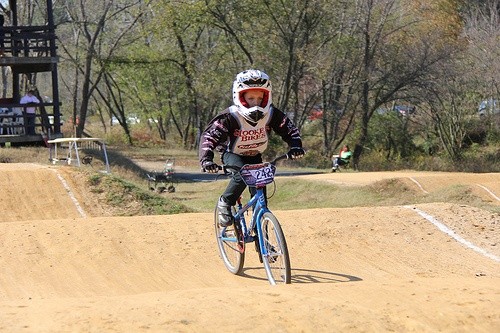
[
  {"left": 331, "top": 144, "right": 351, "bottom": 172},
  {"left": 199, "top": 71, "right": 305, "bottom": 264},
  {"left": 20, "top": 91, "right": 40, "bottom": 135},
  {"left": 0, "top": 14, "right": 4, "bottom": 56}
]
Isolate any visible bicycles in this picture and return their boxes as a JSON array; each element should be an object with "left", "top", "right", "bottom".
[{"left": 201, "top": 149, "right": 292, "bottom": 288}]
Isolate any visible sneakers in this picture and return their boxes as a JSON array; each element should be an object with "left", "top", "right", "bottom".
[
  {"left": 217, "top": 195, "right": 232, "bottom": 226},
  {"left": 260, "top": 250, "right": 276, "bottom": 263}
]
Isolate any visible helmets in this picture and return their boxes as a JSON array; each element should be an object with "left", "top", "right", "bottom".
[{"left": 233, "top": 70, "right": 272, "bottom": 124}]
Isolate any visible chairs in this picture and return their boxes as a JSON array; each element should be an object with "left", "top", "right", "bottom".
[
  {"left": 335, "top": 157, "right": 353, "bottom": 173},
  {"left": 0, "top": 107, "right": 25, "bottom": 135}
]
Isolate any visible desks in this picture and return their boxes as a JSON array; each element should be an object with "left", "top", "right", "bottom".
[{"left": 46, "top": 138, "right": 101, "bottom": 168}]
